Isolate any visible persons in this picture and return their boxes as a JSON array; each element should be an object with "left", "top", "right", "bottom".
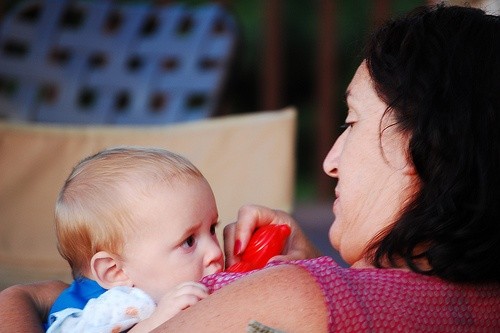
[
  {"left": 2, "top": 6, "right": 500, "bottom": 333},
  {"left": 43, "top": 143, "right": 226, "bottom": 333}
]
